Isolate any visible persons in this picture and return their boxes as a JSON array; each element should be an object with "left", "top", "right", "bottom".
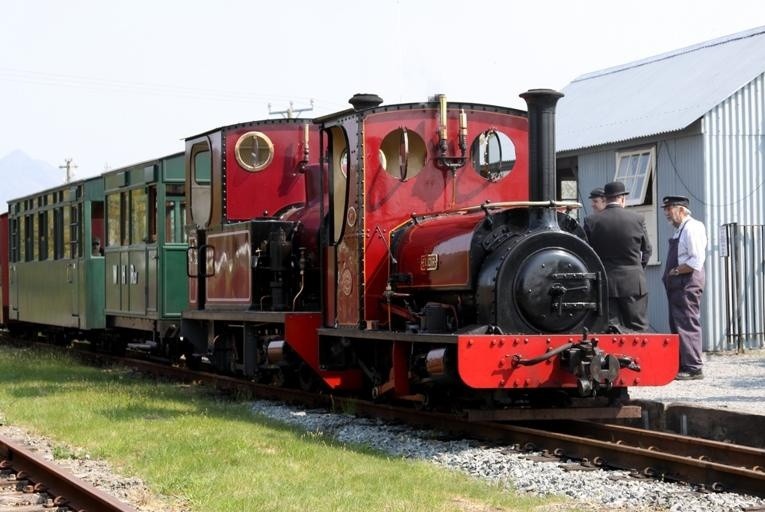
[
  {"left": 583, "top": 181, "right": 652, "bottom": 334},
  {"left": 660, "top": 196, "right": 707, "bottom": 379}
]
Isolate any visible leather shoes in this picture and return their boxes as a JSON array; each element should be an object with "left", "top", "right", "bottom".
[{"left": 677, "top": 367, "right": 705, "bottom": 380}]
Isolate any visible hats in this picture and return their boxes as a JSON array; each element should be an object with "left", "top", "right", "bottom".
[
  {"left": 660, "top": 195, "right": 690, "bottom": 208},
  {"left": 587, "top": 182, "right": 630, "bottom": 199}
]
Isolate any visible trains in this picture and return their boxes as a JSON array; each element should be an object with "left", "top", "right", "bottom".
[{"left": 0, "top": 82, "right": 688, "bottom": 435}]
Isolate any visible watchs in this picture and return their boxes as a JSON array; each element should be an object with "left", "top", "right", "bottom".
[{"left": 675, "top": 267, "right": 680, "bottom": 276}]
